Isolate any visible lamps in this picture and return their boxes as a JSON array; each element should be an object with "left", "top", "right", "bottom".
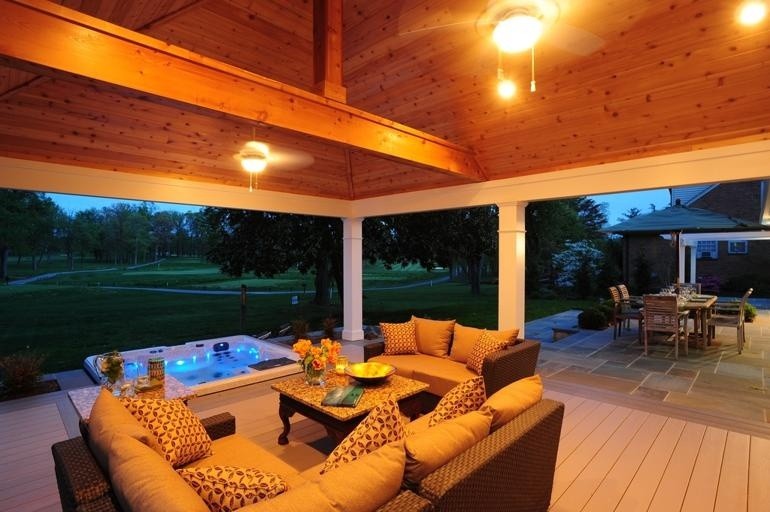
[
  {"left": 231, "top": 122, "right": 276, "bottom": 193},
  {"left": 474, "top": 0, "right": 560, "bottom": 102}
]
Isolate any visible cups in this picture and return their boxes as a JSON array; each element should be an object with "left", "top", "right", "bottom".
[
  {"left": 658, "top": 285, "right": 692, "bottom": 307},
  {"left": 147, "top": 357, "right": 165, "bottom": 382},
  {"left": 335, "top": 355, "right": 349, "bottom": 376}
]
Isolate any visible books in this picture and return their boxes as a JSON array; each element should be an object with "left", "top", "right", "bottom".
[{"left": 320, "top": 386, "right": 365, "bottom": 407}]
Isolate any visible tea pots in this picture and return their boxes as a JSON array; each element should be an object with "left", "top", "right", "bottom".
[{"left": 93, "top": 352, "right": 125, "bottom": 393}]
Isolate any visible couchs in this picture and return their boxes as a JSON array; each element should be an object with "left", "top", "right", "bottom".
[
  {"left": 50, "top": 374, "right": 565, "bottom": 512},
  {"left": 364, "top": 319, "right": 540, "bottom": 409}
]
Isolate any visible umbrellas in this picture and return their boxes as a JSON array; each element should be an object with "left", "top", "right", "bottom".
[{"left": 600, "top": 198, "right": 770, "bottom": 294}]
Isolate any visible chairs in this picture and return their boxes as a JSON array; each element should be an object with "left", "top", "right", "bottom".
[{"left": 608, "top": 283, "right": 753, "bottom": 359}]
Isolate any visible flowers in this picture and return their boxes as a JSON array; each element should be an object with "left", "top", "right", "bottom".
[{"left": 291, "top": 338, "right": 343, "bottom": 377}]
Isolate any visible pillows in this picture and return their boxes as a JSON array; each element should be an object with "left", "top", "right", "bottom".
[
  {"left": 379, "top": 315, "right": 456, "bottom": 359},
  {"left": 450, "top": 323, "right": 519, "bottom": 376}
]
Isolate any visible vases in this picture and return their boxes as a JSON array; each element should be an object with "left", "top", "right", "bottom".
[{"left": 306, "top": 367, "right": 325, "bottom": 386}]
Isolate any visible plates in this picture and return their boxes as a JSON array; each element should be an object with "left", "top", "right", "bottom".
[
  {"left": 132, "top": 376, "right": 162, "bottom": 391},
  {"left": 690, "top": 295, "right": 713, "bottom": 302}
]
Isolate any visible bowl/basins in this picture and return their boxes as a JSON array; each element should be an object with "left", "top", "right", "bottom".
[{"left": 344, "top": 362, "right": 399, "bottom": 384}]
